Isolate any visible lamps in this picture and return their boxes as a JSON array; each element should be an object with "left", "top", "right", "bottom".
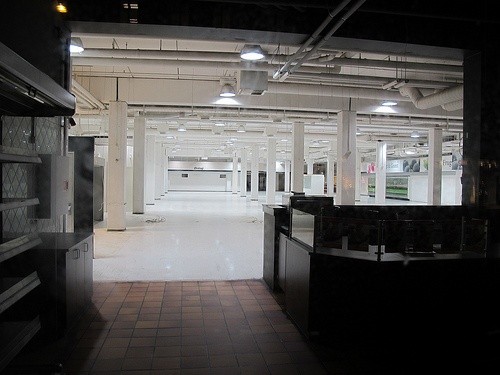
[
  {"left": 68, "top": 37, "right": 85, "bottom": 55},
  {"left": 239, "top": 44, "right": 265, "bottom": 61},
  {"left": 149, "top": 77, "right": 440, "bottom": 159}
]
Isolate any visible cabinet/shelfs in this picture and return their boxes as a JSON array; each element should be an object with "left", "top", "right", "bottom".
[
  {"left": 0, "top": 42, "right": 78, "bottom": 375},
  {"left": 35, "top": 230, "right": 96, "bottom": 322},
  {"left": 32, "top": 149, "right": 72, "bottom": 220}
]
